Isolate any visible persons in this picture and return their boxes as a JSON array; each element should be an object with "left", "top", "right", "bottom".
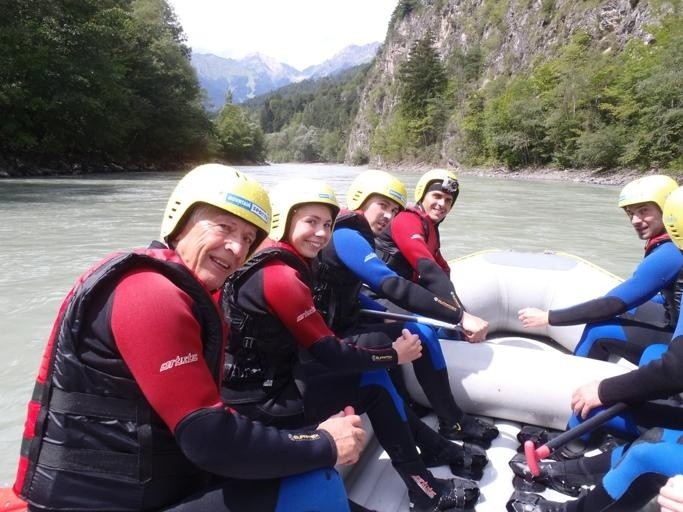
[
  {"left": 517, "top": 174, "right": 683, "bottom": 361},
  {"left": 517, "top": 184, "right": 683, "bottom": 462},
  {"left": 312, "top": 168, "right": 499, "bottom": 450},
  {"left": 218, "top": 177, "right": 489, "bottom": 511},
  {"left": 359, "top": 168, "right": 465, "bottom": 312},
  {"left": 12, "top": 163, "right": 368, "bottom": 511},
  {"left": 506, "top": 427, "right": 682, "bottom": 512}
]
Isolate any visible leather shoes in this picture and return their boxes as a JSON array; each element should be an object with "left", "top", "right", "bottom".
[
  {"left": 438, "top": 410, "right": 500, "bottom": 446},
  {"left": 416, "top": 438, "right": 488, "bottom": 483},
  {"left": 505, "top": 488, "right": 594, "bottom": 512},
  {"left": 517, "top": 424, "right": 586, "bottom": 461},
  {"left": 407, "top": 476, "right": 482, "bottom": 512},
  {"left": 508, "top": 453, "right": 585, "bottom": 497}
]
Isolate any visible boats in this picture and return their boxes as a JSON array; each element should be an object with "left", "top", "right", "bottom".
[{"left": 181, "top": 247, "right": 682, "bottom": 511}]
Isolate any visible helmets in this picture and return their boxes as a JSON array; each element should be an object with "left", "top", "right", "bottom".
[
  {"left": 159, "top": 160, "right": 272, "bottom": 270},
  {"left": 268, "top": 178, "right": 341, "bottom": 242},
  {"left": 413, "top": 168, "right": 461, "bottom": 212},
  {"left": 662, "top": 185, "right": 683, "bottom": 249},
  {"left": 618, "top": 174, "right": 679, "bottom": 233},
  {"left": 347, "top": 170, "right": 408, "bottom": 219}
]
